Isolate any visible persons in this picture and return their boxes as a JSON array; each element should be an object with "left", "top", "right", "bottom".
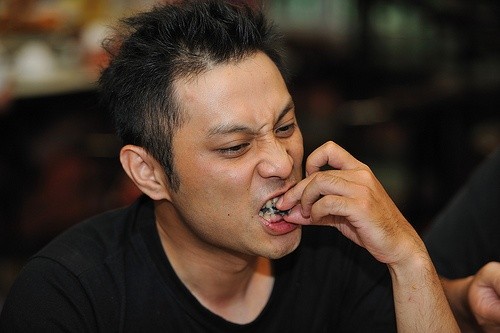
[
  {"left": 0, "top": 0, "right": 461, "bottom": 333},
  {"left": 418, "top": 143, "right": 500, "bottom": 333}
]
